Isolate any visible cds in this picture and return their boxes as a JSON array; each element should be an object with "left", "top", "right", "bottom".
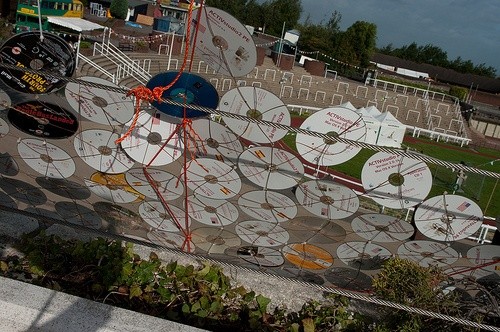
[{"left": 0, "top": 6, "right": 500, "bottom": 308}]
